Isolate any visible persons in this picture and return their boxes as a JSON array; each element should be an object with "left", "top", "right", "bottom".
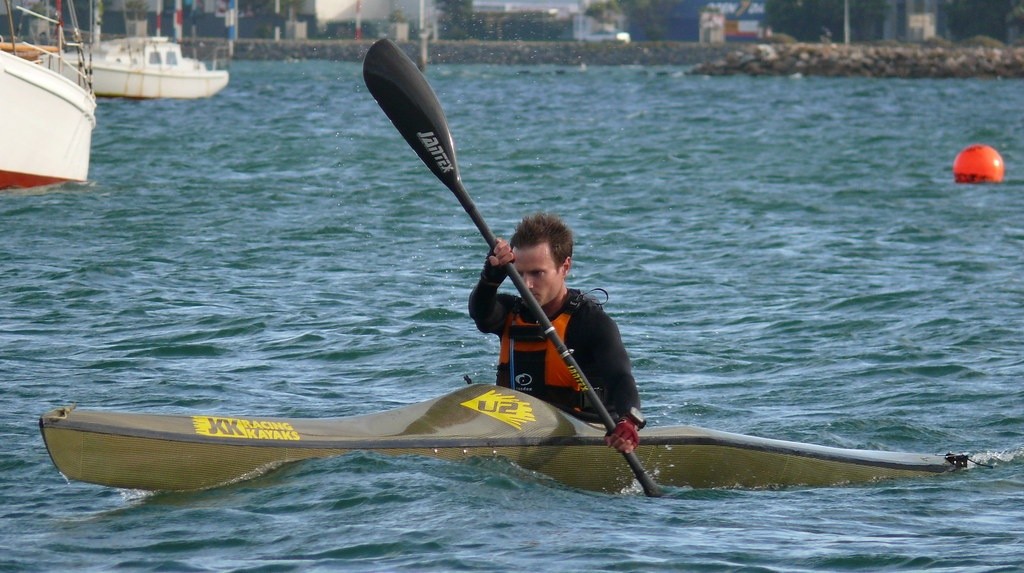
[{"left": 467, "top": 211, "right": 646, "bottom": 455}]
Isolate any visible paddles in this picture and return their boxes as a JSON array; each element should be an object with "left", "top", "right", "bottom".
[{"left": 363, "top": 36, "right": 667, "bottom": 496}]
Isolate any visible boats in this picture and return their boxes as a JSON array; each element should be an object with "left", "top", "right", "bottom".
[
  {"left": 36, "top": 385, "right": 974, "bottom": 495},
  {"left": 0, "top": 41, "right": 60, "bottom": 61},
  {"left": 37, "top": 35, "right": 230, "bottom": 99},
  {"left": 0, "top": 0, "right": 97, "bottom": 188}
]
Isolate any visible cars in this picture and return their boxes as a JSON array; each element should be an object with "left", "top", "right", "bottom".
[{"left": 579, "top": 26, "right": 631, "bottom": 45}]
